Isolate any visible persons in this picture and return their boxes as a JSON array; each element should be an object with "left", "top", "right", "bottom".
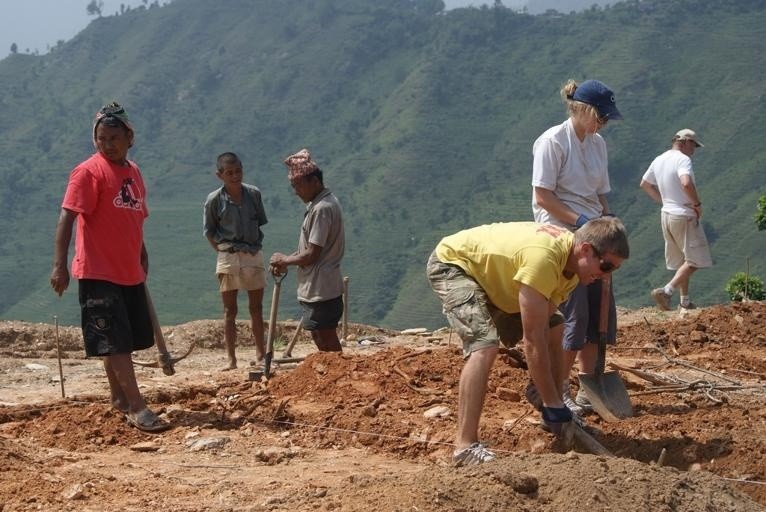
[
  {"left": 426, "top": 217, "right": 630, "bottom": 467},
  {"left": 203, "top": 152, "right": 269, "bottom": 371},
  {"left": 640, "top": 128, "right": 713, "bottom": 311},
  {"left": 531, "top": 80, "right": 624, "bottom": 417},
  {"left": 268, "top": 148, "right": 346, "bottom": 352},
  {"left": 50, "top": 101, "right": 170, "bottom": 432}
]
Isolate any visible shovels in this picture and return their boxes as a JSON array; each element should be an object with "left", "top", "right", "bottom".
[
  {"left": 578, "top": 213, "right": 633, "bottom": 423},
  {"left": 249, "top": 266, "right": 288, "bottom": 382}
]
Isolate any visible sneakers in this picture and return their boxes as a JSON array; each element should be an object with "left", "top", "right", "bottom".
[
  {"left": 651, "top": 287, "right": 674, "bottom": 311},
  {"left": 678, "top": 303, "right": 699, "bottom": 311},
  {"left": 541, "top": 373, "right": 594, "bottom": 431},
  {"left": 452, "top": 443, "right": 498, "bottom": 468}
]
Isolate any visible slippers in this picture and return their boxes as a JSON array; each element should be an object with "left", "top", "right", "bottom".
[{"left": 125, "top": 407, "right": 170, "bottom": 431}]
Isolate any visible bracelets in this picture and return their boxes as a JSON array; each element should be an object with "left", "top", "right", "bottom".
[
  {"left": 694, "top": 203, "right": 702, "bottom": 207},
  {"left": 576, "top": 213, "right": 589, "bottom": 228}
]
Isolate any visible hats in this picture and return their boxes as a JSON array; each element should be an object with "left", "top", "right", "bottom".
[
  {"left": 573, "top": 80, "right": 624, "bottom": 120},
  {"left": 675, "top": 129, "right": 704, "bottom": 147},
  {"left": 285, "top": 149, "right": 318, "bottom": 180}
]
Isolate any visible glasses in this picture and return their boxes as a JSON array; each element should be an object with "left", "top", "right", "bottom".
[{"left": 591, "top": 244, "right": 620, "bottom": 272}]
